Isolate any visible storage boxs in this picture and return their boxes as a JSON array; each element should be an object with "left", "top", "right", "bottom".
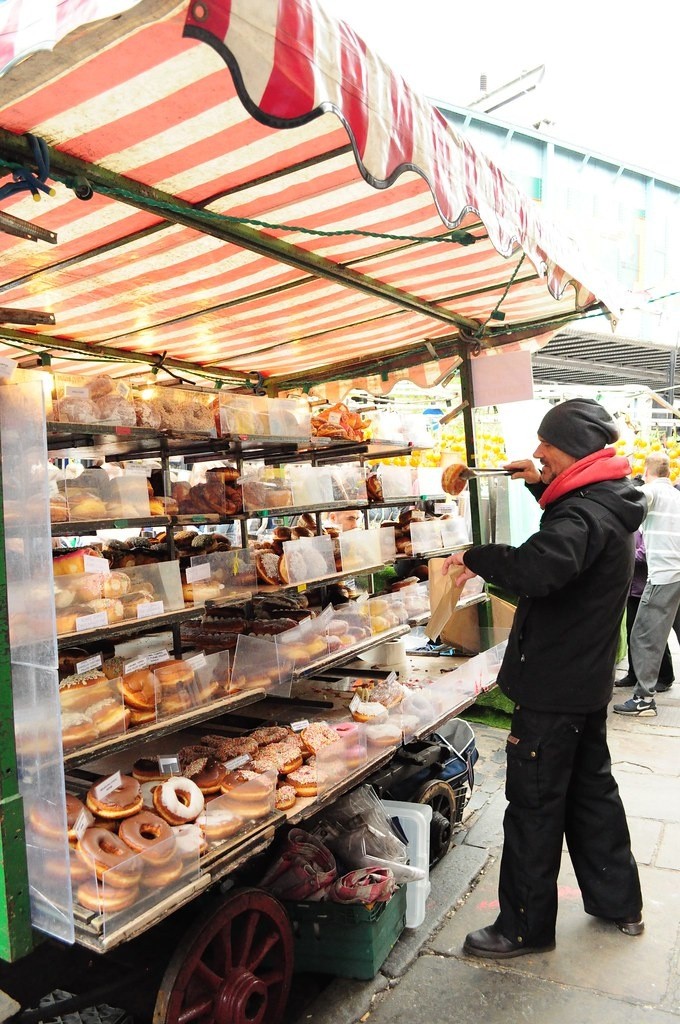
[{"left": 275, "top": 882, "right": 414, "bottom": 977}]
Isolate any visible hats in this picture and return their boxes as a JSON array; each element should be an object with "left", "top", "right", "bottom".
[{"left": 536, "top": 398, "right": 620, "bottom": 459}]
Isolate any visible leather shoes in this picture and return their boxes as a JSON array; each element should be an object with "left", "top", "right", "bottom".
[
  {"left": 613, "top": 911, "right": 644, "bottom": 935},
  {"left": 463, "top": 925, "right": 556, "bottom": 959}
]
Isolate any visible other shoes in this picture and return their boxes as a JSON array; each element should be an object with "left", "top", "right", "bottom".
[
  {"left": 653, "top": 682, "right": 672, "bottom": 691},
  {"left": 614, "top": 676, "right": 637, "bottom": 687}
]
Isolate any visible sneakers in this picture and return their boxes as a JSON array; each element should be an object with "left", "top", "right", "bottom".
[{"left": 613, "top": 695, "right": 658, "bottom": 716}]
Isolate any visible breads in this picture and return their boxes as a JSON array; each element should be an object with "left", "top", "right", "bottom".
[
  {"left": 443, "top": 463, "right": 468, "bottom": 495},
  {"left": 13, "top": 509, "right": 463, "bottom": 757}
]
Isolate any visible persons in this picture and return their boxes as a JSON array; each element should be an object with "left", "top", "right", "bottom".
[
  {"left": 613, "top": 452, "right": 680, "bottom": 718},
  {"left": 616, "top": 474, "right": 675, "bottom": 694},
  {"left": 445, "top": 398, "right": 645, "bottom": 961}
]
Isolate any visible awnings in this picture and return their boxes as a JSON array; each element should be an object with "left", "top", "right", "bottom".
[{"left": 0, "top": 0, "right": 632, "bottom": 452}]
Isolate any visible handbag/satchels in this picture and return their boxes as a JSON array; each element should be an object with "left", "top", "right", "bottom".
[{"left": 382, "top": 718, "right": 480, "bottom": 809}]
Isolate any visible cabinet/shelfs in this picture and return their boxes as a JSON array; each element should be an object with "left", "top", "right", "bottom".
[{"left": 1, "top": 326, "right": 498, "bottom": 955}]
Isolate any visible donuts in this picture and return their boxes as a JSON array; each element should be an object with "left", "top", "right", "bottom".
[
  {"left": 3, "top": 374, "right": 385, "bottom": 524},
  {"left": 30, "top": 682, "right": 436, "bottom": 912}
]
